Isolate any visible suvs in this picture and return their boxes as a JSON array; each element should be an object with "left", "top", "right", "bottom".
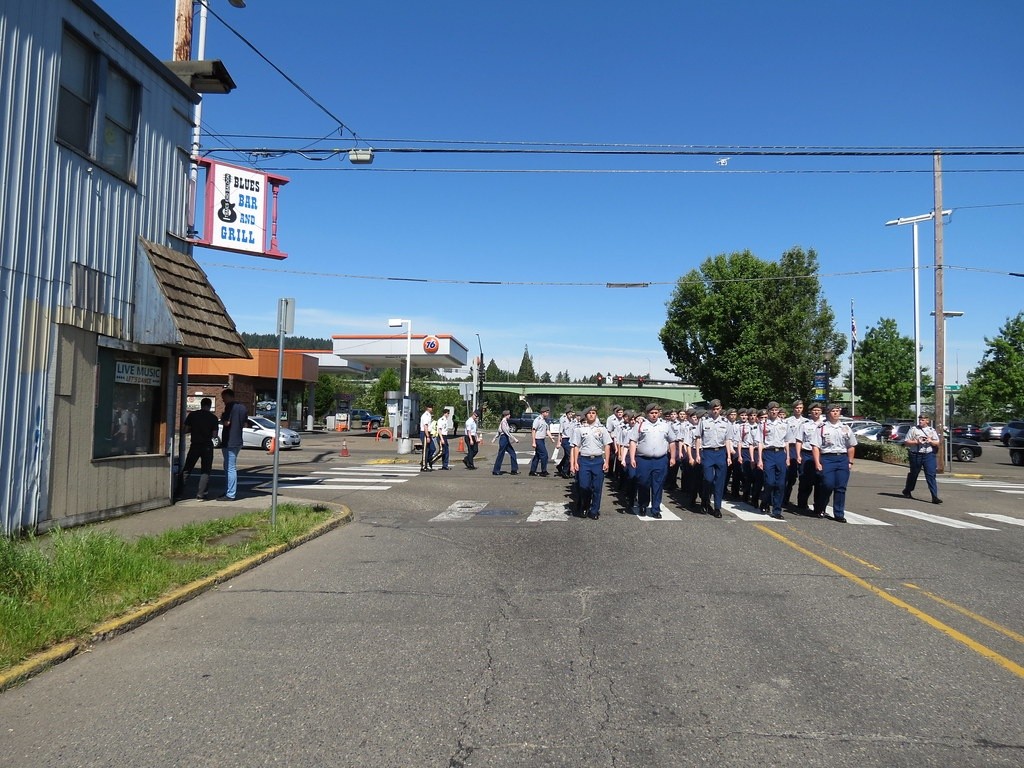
[
  {"left": 350, "top": 409, "right": 385, "bottom": 429},
  {"left": 876, "top": 422, "right": 982, "bottom": 462},
  {"left": 1000, "top": 421, "right": 1024, "bottom": 466}
]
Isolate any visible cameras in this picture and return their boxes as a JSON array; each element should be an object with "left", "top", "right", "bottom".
[{"left": 922, "top": 437, "right": 927, "bottom": 441}]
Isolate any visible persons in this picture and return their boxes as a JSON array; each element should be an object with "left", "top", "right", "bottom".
[
  {"left": 902, "top": 413, "right": 943, "bottom": 504},
  {"left": 462, "top": 409, "right": 481, "bottom": 470},
  {"left": 490, "top": 409, "right": 521, "bottom": 475},
  {"left": 556, "top": 399, "right": 858, "bottom": 523},
  {"left": 113, "top": 398, "right": 137, "bottom": 441},
  {"left": 182, "top": 389, "right": 248, "bottom": 501},
  {"left": 452, "top": 415, "right": 459, "bottom": 438},
  {"left": 419, "top": 403, "right": 452, "bottom": 472},
  {"left": 528, "top": 407, "right": 555, "bottom": 477}
]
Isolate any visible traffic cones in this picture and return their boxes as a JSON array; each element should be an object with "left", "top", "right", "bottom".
[
  {"left": 339, "top": 438, "right": 351, "bottom": 457},
  {"left": 267, "top": 438, "right": 275, "bottom": 455}
]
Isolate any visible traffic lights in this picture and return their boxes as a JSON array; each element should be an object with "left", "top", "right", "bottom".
[
  {"left": 618, "top": 376, "right": 622, "bottom": 387},
  {"left": 638, "top": 377, "right": 643, "bottom": 388},
  {"left": 598, "top": 376, "right": 602, "bottom": 386}
]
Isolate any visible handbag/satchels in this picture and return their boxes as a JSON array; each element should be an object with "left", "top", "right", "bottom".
[{"left": 918, "top": 425, "right": 938, "bottom": 454}]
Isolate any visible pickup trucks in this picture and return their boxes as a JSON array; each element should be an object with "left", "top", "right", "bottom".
[{"left": 501, "top": 412, "right": 553, "bottom": 433}]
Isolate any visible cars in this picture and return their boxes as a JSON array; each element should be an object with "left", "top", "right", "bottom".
[
  {"left": 839, "top": 414, "right": 881, "bottom": 441},
  {"left": 211, "top": 416, "right": 301, "bottom": 450},
  {"left": 952, "top": 423, "right": 981, "bottom": 442},
  {"left": 980, "top": 422, "right": 1006, "bottom": 440}
]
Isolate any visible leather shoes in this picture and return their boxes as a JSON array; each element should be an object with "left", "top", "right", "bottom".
[
  {"left": 760, "top": 504, "right": 784, "bottom": 519},
  {"left": 814, "top": 504, "right": 846, "bottom": 523},
  {"left": 529, "top": 471, "right": 549, "bottom": 477},
  {"left": 640, "top": 507, "right": 662, "bottom": 518},
  {"left": 581, "top": 510, "right": 598, "bottom": 520},
  {"left": 701, "top": 506, "right": 722, "bottom": 518}
]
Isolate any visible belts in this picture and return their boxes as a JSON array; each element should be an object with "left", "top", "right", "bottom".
[
  {"left": 706, "top": 448, "right": 722, "bottom": 451},
  {"left": 825, "top": 453, "right": 846, "bottom": 456},
  {"left": 734, "top": 448, "right": 784, "bottom": 452},
  {"left": 584, "top": 456, "right": 602, "bottom": 459},
  {"left": 641, "top": 454, "right": 666, "bottom": 460}
]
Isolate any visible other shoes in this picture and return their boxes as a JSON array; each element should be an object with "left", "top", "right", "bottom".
[
  {"left": 902, "top": 489, "right": 913, "bottom": 498},
  {"left": 797, "top": 504, "right": 808, "bottom": 512},
  {"left": 420, "top": 468, "right": 452, "bottom": 472},
  {"left": 463, "top": 460, "right": 475, "bottom": 469},
  {"left": 932, "top": 497, "right": 942, "bottom": 503},
  {"left": 196, "top": 496, "right": 209, "bottom": 502},
  {"left": 493, "top": 472, "right": 519, "bottom": 475},
  {"left": 556, "top": 465, "right": 569, "bottom": 479},
  {"left": 743, "top": 497, "right": 758, "bottom": 508},
  {"left": 216, "top": 495, "right": 235, "bottom": 501}
]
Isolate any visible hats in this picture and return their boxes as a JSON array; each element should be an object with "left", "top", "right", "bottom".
[
  {"left": 425, "top": 404, "right": 550, "bottom": 416},
  {"left": 564, "top": 399, "right": 840, "bottom": 422},
  {"left": 918, "top": 414, "right": 928, "bottom": 418}
]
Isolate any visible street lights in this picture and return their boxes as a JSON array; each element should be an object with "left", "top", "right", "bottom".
[
  {"left": 930, "top": 311, "right": 964, "bottom": 426},
  {"left": 885, "top": 210, "right": 952, "bottom": 424},
  {"left": 388, "top": 319, "right": 411, "bottom": 438}
]
